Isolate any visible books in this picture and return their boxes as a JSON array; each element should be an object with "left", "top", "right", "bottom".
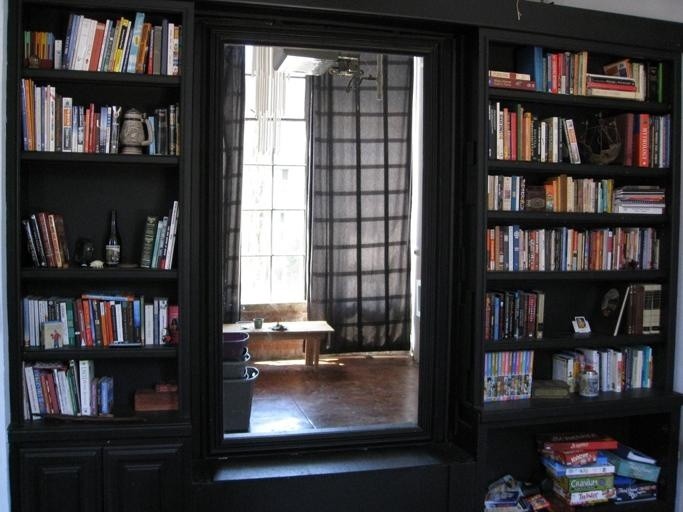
[
  {"left": 14, "top": 9, "right": 181, "bottom": 156},
  {"left": 25, "top": 200, "right": 180, "bottom": 271},
  {"left": 535, "top": 427, "right": 660, "bottom": 506},
  {"left": 485, "top": 346, "right": 653, "bottom": 402},
  {"left": 485, "top": 283, "right": 662, "bottom": 343},
  {"left": 487, "top": 175, "right": 664, "bottom": 272},
  {"left": 21, "top": 290, "right": 182, "bottom": 425},
  {"left": 487, "top": 43, "right": 670, "bottom": 168}
]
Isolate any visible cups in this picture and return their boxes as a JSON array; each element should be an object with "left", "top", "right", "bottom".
[{"left": 253, "top": 318, "right": 264, "bottom": 329}]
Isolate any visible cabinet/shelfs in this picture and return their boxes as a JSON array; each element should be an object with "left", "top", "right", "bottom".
[
  {"left": 451, "top": 26, "right": 680, "bottom": 418},
  {"left": 9, "top": 433, "right": 202, "bottom": 511},
  {"left": 6, "top": 0, "right": 196, "bottom": 431}
]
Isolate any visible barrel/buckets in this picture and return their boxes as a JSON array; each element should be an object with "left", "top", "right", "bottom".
[{"left": 223, "top": 331, "right": 260, "bottom": 434}]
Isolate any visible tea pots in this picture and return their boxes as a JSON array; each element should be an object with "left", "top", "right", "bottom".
[{"left": 117, "top": 108, "right": 154, "bottom": 156}]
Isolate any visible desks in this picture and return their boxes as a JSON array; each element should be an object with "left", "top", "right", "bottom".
[{"left": 221, "top": 319, "right": 337, "bottom": 375}]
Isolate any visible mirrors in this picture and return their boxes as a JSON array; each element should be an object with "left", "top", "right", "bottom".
[{"left": 196, "top": 17, "right": 444, "bottom": 453}]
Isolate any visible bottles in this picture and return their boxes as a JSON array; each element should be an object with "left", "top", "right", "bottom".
[
  {"left": 579, "top": 366, "right": 600, "bottom": 398},
  {"left": 104, "top": 209, "right": 120, "bottom": 266}
]
